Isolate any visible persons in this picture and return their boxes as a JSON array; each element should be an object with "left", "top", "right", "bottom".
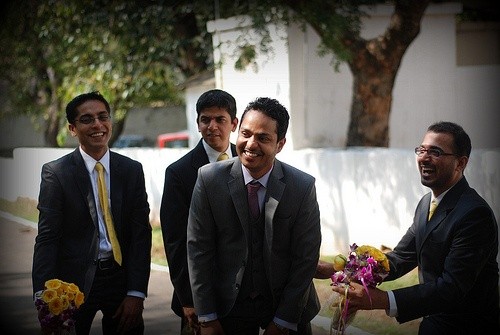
[
  {"left": 32, "top": 90, "right": 153, "bottom": 335},
  {"left": 314, "top": 121, "right": 500, "bottom": 335},
  {"left": 160, "top": 89, "right": 323, "bottom": 335}
]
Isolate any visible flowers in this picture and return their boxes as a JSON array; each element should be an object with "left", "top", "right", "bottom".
[
  {"left": 33, "top": 279, "right": 85, "bottom": 335},
  {"left": 330, "top": 243, "right": 390, "bottom": 335}
]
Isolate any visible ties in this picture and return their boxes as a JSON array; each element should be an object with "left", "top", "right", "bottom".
[
  {"left": 218, "top": 153, "right": 229, "bottom": 161},
  {"left": 246, "top": 182, "right": 263, "bottom": 300},
  {"left": 95, "top": 162, "right": 122, "bottom": 266},
  {"left": 428, "top": 200, "right": 438, "bottom": 221}
]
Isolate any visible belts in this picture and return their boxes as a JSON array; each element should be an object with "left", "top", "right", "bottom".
[{"left": 95, "top": 259, "right": 119, "bottom": 270}]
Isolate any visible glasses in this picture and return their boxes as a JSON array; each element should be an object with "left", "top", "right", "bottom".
[
  {"left": 415, "top": 146, "right": 463, "bottom": 159},
  {"left": 73, "top": 114, "right": 111, "bottom": 124}
]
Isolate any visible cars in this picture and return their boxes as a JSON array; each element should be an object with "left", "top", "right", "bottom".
[
  {"left": 111, "top": 134, "right": 155, "bottom": 148},
  {"left": 156, "top": 132, "right": 188, "bottom": 149}
]
